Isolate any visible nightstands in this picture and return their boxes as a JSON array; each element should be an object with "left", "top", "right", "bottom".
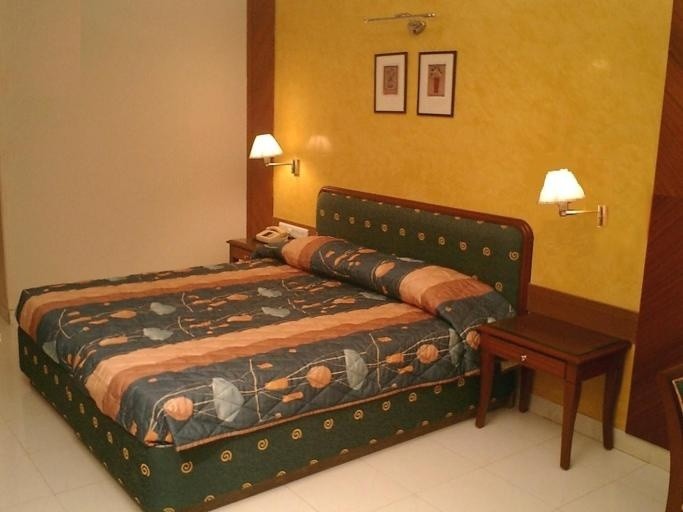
[
  {"left": 226, "top": 236, "right": 270, "bottom": 264},
  {"left": 473, "top": 312, "right": 632, "bottom": 472}
]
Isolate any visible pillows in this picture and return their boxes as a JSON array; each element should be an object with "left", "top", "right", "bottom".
[{"left": 252, "top": 234, "right": 515, "bottom": 369}]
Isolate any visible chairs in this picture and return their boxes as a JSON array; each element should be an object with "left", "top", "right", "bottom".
[{"left": 655, "top": 359, "right": 683, "bottom": 512}]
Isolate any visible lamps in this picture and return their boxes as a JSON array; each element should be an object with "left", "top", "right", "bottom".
[
  {"left": 537, "top": 168, "right": 607, "bottom": 228},
  {"left": 249, "top": 133, "right": 299, "bottom": 176}
]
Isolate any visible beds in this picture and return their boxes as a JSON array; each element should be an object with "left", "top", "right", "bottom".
[{"left": 15, "top": 186, "right": 534, "bottom": 512}]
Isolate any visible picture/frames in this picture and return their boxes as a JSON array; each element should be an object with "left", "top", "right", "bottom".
[
  {"left": 417, "top": 50, "right": 456, "bottom": 117},
  {"left": 374, "top": 52, "right": 407, "bottom": 113}
]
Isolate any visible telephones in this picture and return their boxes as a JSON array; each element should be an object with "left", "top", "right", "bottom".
[{"left": 256, "top": 226, "right": 290, "bottom": 245}]
